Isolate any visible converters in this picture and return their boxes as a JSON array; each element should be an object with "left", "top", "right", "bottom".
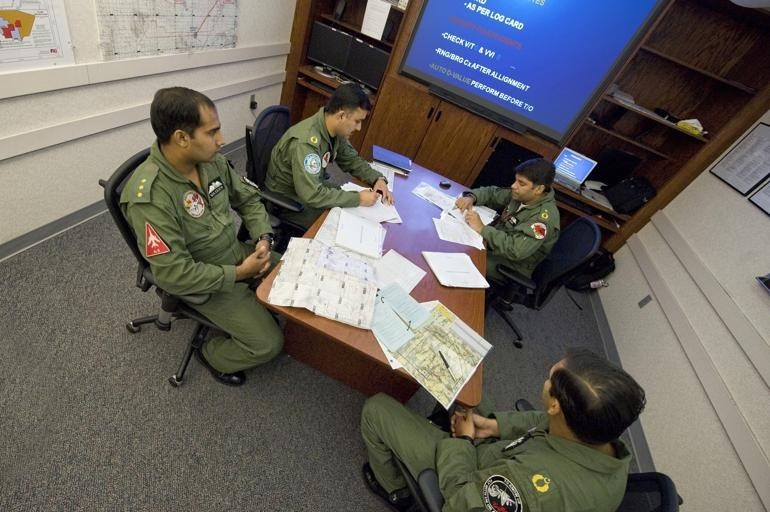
[{"left": 251, "top": 101, "right": 257, "bottom": 109}]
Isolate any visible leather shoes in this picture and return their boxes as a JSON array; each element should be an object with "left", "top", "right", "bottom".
[
  {"left": 362, "top": 461, "right": 415, "bottom": 510},
  {"left": 192, "top": 340, "right": 246, "bottom": 386}
]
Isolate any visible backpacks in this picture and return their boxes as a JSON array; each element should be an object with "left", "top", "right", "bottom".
[{"left": 563, "top": 246, "right": 616, "bottom": 312}]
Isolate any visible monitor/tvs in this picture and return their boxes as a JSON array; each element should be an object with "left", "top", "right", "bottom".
[
  {"left": 340, "top": 37, "right": 391, "bottom": 95},
  {"left": 593, "top": 143, "right": 640, "bottom": 187},
  {"left": 305, "top": 19, "right": 354, "bottom": 79}
]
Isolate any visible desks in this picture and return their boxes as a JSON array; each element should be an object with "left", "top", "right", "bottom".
[{"left": 251, "top": 136, "right": 496, "bottom": 421}]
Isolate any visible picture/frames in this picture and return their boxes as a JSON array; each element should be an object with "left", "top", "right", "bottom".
[
  {"left": 707, "top": 120, "right": 770, "bottom": 197},
  {"left": 746, "top": 180, "right": 769, "bottom": 218}
]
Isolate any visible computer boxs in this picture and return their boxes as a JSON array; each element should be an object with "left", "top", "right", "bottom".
[{"left": 565, "top": 245, "right": 615, "bottom": 294}]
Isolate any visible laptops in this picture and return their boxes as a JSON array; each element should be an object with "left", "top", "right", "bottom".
[{"left": 552, "top": 146, "right": 599, "bottom": 195}]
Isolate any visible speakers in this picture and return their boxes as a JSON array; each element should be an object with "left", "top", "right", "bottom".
[{"left": 605, "top": 175, "right": 657, "bottom": 214}]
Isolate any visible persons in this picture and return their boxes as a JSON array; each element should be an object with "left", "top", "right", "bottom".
[
  {"left": 360, "top": 344, "right": 647, "bottom": 511},
  {"left": 119, "top": 85, "right": 287, "bottom": 387},
  {"left": 263, "top": 82, "right": 394, "bottom": 231},
  {"left": 455, "top": 157, "right": 561, "bottom": 300}
]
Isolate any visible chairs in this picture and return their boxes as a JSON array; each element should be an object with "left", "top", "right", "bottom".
[
  {"left": 616, "top": 470, "right": 684, "bottom": 512},
  {"left": 96, "top": 145, "right": 237, "bottom": 389},
  {"left": 235, "top": 105, "right": 306, "bottom": 252},
  {"left": 483, "top": 211, "right": 605, "bottom": 350}
]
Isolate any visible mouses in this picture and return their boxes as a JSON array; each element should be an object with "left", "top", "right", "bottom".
[{"left": 440, "top": 180, "right": 453, "bottom": 188}]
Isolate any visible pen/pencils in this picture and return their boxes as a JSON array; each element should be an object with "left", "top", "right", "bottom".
[{"left": 439, "top": 351, "right": 457, "bottom": 381}]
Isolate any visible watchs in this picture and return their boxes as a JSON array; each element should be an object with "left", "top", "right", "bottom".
[
  {"left": 464, "top": 192, "right": 477, "bottom": 203},
  {"left": 254, "top": 235, "right": 276, "bottom": 251},
  {"left": 377, "top": 176, "right": 390, "bottom": 186}
]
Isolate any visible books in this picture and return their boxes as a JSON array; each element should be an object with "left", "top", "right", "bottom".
[{"left": 611, "top": 89, "right": 636, "bottom": 104}]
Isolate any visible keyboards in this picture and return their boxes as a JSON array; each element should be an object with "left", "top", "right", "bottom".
[
  {"left": 580, "top": 189, "right": 617, "bottom": 216},
  {"left": 310, "top": 78, "right": 336, "bottom": 95},
  {"left": 553, "top": 191, "right": 598, "bottom": 216}
]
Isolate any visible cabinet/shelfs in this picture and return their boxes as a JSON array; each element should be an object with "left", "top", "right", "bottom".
[
  {"left": 464, "top": 122, "right": 556, "bottom": 196},
  {"left": 539, "top": 2, "right": 769, "bottom": 253},
  {"left": 358, "top": 72, "right": 498, "bottom": 189},
  {"left": 282, "top": 0, "right": 426, "bottom": 153}
]
